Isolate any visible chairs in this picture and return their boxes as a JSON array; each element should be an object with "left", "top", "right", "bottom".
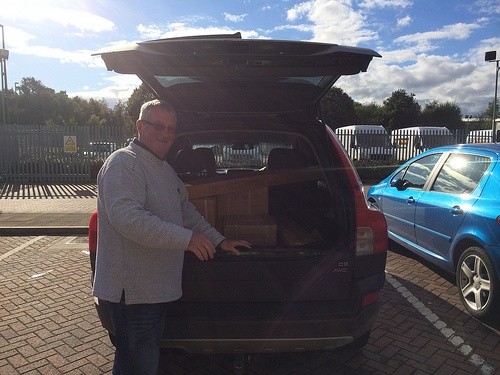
[
  {"left": 177, "top": 147, "right": 216, "bottom": 179},
  {"left": 267, "top": 148, "right": 308, "bottom": 172}
]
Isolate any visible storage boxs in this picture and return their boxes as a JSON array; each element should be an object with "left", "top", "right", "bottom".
[{"left": 188, "top": 187, "right": 279, "bottom": 248}]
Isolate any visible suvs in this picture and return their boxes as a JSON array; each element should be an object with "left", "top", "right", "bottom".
[{"left": 88, "top": 32, "right": 388, "bottom": 364}]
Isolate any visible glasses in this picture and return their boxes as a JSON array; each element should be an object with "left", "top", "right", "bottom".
[{"left": 140, "top": 119, "right": 178, "bottom": 133}]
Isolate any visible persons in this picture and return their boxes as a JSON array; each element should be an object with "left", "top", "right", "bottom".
[{"left": 92, "top": 100, "right": 254, "bottom": 375}]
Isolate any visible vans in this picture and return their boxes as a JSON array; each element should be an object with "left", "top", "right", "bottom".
[
  {"left": 391, "top": 127, "right": 454, "bottom": 161},
  {"left": 465, "top": 130, "right": 499, "bottom": 143},
  {"left": 335, "top": 125, "right": 396, "bottom": 162}
]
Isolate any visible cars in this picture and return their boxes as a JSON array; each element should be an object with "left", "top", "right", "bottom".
[
  {"left": 367, "top": 142, "right": 500, "bottom": 320},
  {"left": 82, "top": 142, "right": 116, "bottom": 161}
]
